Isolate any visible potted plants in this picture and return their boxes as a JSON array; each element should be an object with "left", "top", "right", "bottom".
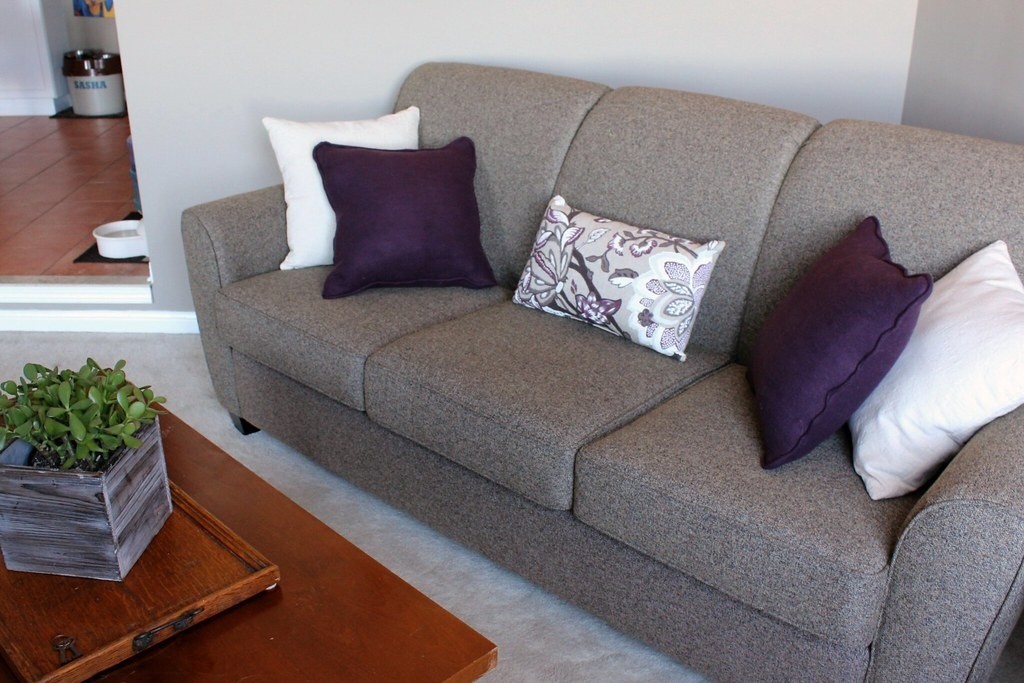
[{"left": 0, "top": 358, "right": 171, "bottom": 585}]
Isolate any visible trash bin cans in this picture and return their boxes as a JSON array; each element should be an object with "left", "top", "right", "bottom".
[{"left": 62, "top": 47, "right": 126, "bottom": 116}]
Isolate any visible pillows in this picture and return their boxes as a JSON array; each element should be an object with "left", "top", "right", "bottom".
[
  {"left": 258, "top": 102, "right": 422, "bottom": 273},
  {"left": 848, "top": 239, "right": 1024, "bottom": 499},
  {"left": 310, "top": 137, "right": 495, "bottom": 301},
  {"left": 512, "top": 195, "right": 728, "bottom": 362},
  {"left": 748, "top": 216, "right": 934, "bottom": 476}
]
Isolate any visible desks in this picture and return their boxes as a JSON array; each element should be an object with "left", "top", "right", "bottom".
[{"left": 1, "top": 368, "right": 500, "bottom": 683}]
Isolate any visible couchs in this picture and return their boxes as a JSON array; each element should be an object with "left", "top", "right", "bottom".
[{"left": 179, "top": 58, "right": 1022, "bottom": 682}]
